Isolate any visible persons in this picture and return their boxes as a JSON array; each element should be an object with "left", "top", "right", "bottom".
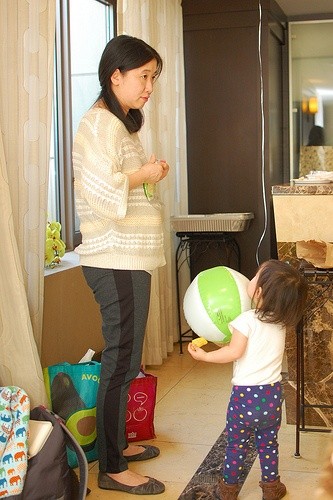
[
  {"left": 186, "top": 260, "right": 308, "bottom": 500},
  {"left": 73, "top": 35, "right": 169, "bottom": 495}
]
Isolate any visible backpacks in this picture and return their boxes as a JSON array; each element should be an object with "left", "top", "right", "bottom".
[{"left": 20, "top": 405, "right": 92, "bottom": 500}]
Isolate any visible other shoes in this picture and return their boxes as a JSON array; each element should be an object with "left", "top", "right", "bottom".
[
  {"left": 97, "top": 469, "right": 165, "bottom": 496},
  {"left": 259, "top": 475, "right": 286, "bottom": 500},
  {"left": 121, "top": 444, "right": 161, "bottom": 462},
  {"left": 216, "top": 478, "right": 238, "bottom": 500}
]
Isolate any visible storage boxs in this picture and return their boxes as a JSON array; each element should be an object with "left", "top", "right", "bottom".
[{"left": 172, "top": 212, "right": 255, "bottom": 233}]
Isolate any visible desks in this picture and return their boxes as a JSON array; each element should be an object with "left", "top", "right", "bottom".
[{"left": 175, "top": 232, "right": 241, "bottom": 356}]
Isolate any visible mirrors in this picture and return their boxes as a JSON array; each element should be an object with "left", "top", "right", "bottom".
[{"left": 280, "top": 12, "right": 333, "bottom": 186}]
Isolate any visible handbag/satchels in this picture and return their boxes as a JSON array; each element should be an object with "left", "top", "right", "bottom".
[
  {"left": 44, "top": 355, "right": 104, "bottom": 467},
  {"left": 126, "top": 364, "right": 161, "bottom": 443}
]
